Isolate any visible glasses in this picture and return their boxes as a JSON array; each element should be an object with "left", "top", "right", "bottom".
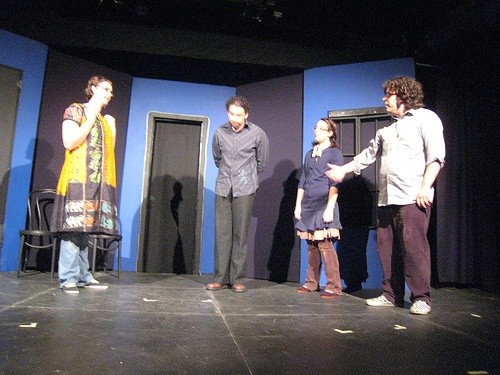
[
  {"left": 97, "top": 84, "right": 114, "bottom": 92},
  {"left": 386, "top": 91, "right": 398, "bottom": 97},
  {"left": 313, "top": 126, "right": 328, "bottom": 131}
]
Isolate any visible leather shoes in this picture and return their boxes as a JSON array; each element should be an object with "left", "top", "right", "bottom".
[
  {"left": 206, "top": 281, "right": 227, "bottom": 290},
  {"left": 231, "top": 283, "right": 246, "bottom": 292}
]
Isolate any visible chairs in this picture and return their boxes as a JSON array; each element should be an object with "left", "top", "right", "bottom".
[{"left": 16, "top": 188, "right": 122, "bottom": 279}]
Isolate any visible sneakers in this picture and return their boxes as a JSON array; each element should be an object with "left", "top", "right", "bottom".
[
  {"left": 85, "top": 279, "right": 110, "bottom": 288},
  {"left": 320, "top": 289, "right": 338, "bottom": 298},
  {"left": 410, "top": 299, "right": 431, "bottom": 315},
  {"left": 365, "top": 293, "right": 400, "bottom": 307},
  {"left": 296, "top": 286, "right": 312, "bottom": 292},
  {"left": 62, "top": 281, "right": 80, "bottom": 293}
]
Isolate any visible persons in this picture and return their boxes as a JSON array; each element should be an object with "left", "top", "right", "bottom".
[
  {"left": 325, "top": 75, "right": 446, "bottom": 315},
  {"left": 206, "top": 96, "right": 270, "bottom": 294},
  {"left": 294, "top": 117, "right": 345, "bottom": 298},
  {"left": 50, "top": 75, "right": 123, "bottom": 294}
]
